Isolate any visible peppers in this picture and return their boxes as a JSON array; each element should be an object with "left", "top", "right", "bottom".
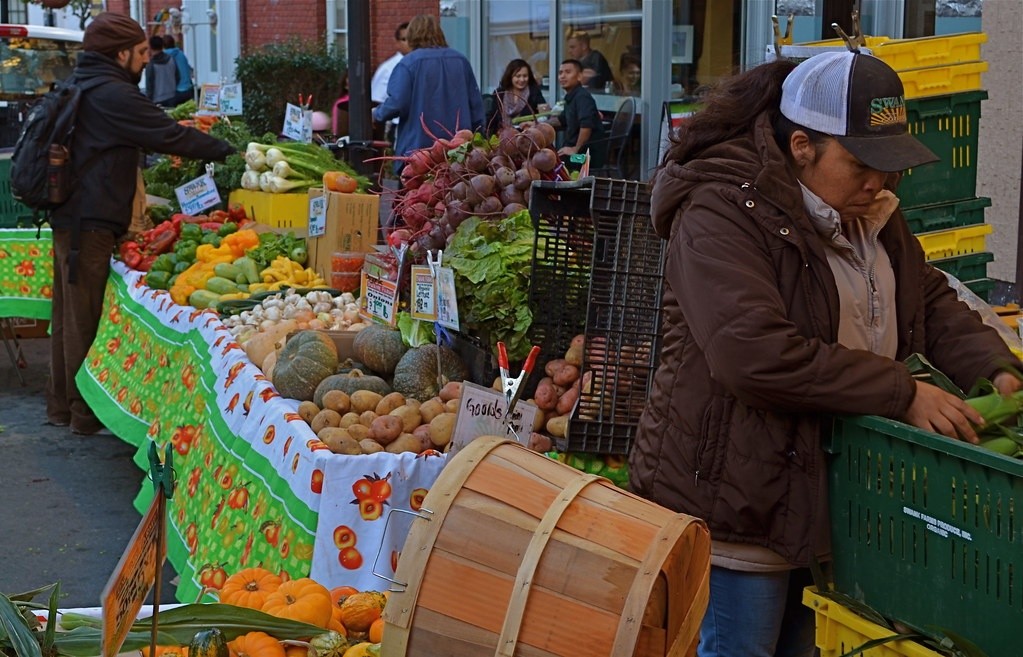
[{"left": 113, "top": 205, "right": 259, "bottom": 304}]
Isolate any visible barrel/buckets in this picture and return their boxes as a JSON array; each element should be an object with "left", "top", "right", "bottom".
[{"left": 373, "top": 434, "right": 712, "bottom": 656}]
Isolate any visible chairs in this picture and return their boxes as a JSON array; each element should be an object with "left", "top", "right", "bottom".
[{"left": 583, "top": 97, "right": 636, "bottom": 180}]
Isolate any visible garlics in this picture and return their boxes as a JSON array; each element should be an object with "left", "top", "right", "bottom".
[{"left": 221, "top": 291, "right": 360, "bottom": 334}]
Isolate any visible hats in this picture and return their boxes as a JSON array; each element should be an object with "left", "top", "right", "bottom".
[
  {"left": 777, "top": 50, "right": 941, "bottom": 174},
  {"left": 84, "top": 11, "right": 146, "bottom": 55}
]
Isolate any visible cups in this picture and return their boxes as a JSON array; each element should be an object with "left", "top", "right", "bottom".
[{"left": 537, "top": 104, "right": 549, "bottom": 113}]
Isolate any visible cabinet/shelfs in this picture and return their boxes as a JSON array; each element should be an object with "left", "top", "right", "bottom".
[{"left": 0, "top": 218, "right": 634, "bottom": 657}]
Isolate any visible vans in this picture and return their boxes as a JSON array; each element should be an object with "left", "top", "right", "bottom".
[{"left": 1, "top": 22, "right": 85, "bottom": 150}]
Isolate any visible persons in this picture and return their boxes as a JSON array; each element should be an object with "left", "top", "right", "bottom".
[
  {"left": 626, "top": 48, "right": 1023, "bottom": 657},
  {"left": 493, "top": 58, "right": 552, "bottom": 134},
  {"left": 610, "top": 52, "right": 642, "bottom": 97},
  {"left": 371, "top": 20, "right": 413, "bottom": 152},
  {"left": 47, "top": 12, "right": 244, "bottom": 435},
  {"left": 371, "top": 12, "right": 488, "bottom": 225},
  {"left": 145, "top": 34, "right": 195, "bottom": 109},
  {"left": 520, "top": 59, "right": 606, "bottom": 171},
  {"left": 566, "top": 30, "right": 614, "bottom": 89}
]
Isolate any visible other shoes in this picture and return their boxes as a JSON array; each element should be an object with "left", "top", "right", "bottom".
[
  {"left": 47, "top": 403, "right": 71, "bottom": 426},
  {"left": 71, "top": 412, "right": 105, "bottom": 435}
]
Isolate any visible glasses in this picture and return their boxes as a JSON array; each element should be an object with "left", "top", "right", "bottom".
[{"left": 396, "top": 37, "right": 408, "bottom": 42}]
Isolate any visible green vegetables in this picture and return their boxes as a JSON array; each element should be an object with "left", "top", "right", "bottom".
[
  {"left": 394, "top": 208, "right": 592, "bottom": 362},
  {"left": 244, "top": 231, "right": 309, "bottom": 266},
  {"left": 141, "top": 120, "right": 375, "bottom": 211},
  {"left": 168, "top": 99, "right": 198, "bottom": 117}
]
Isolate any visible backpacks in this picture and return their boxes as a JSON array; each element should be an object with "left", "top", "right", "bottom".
[{"left": 10, "top": 71, "right": 121, "bottom": 240}]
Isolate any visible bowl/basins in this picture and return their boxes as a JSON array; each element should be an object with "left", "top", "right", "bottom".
[
  {"left": 330, "top": 252, "right": 364, "bottom": 272},
  {"left": 330, "top": 272, "right": 359, "bottom": 291}
]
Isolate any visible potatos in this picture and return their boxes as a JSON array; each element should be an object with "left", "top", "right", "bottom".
[
  {"left": 486, "top": 333, "right": 652, "bottom": 452},
  {"left": 297, "top": 381, "right": 466, "bottom": 455}
]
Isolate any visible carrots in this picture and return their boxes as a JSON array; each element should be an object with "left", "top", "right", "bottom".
[{"left": 169, "top": 113, "right": 219, "bottom": 166}]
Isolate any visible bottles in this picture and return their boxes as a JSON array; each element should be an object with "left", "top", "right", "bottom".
[
  {"left": 605, "top": 81, "right": 609, "bottom": 93},
  {"left": 609, "top": 82, "right": 615, "bottom": 93}
]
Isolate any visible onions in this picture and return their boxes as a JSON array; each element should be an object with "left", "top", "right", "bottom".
[{"left": 235, "top": 307, "right": 367, "bottom": 348}]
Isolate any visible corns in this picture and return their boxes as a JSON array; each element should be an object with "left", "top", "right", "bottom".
[{"left": 953, "top": 390, "right": 1023, "bottom": 459}]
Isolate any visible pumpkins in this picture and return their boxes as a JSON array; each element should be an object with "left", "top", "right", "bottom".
[
  {"left": 335, "top": 175, "right": 357, "bottom": 193},
  {"left": 323, "top": 171, "right": 347, "bottom": 191},
  {"left": 138, "top": 566, "right": 391, "bottom": 657},
  {"left": 244, "top": 318, "right": 470, "bottom": 408}
]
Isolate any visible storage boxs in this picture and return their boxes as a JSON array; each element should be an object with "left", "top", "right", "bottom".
[
  {"left": 439, "top": 32, "right": 1022, "bottom": 657},
  {"left": 0, "top": 159, "right": 46, "bottom": 229},
  {"left": 307, "top": 188, "right": 379, "bottom": 287},
  {"left": 229, "top": 188, "right": 308, "bottom": 229}
]
